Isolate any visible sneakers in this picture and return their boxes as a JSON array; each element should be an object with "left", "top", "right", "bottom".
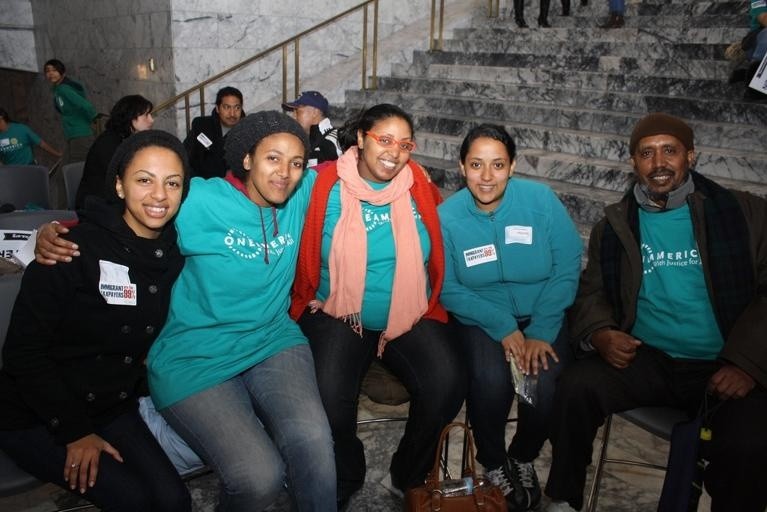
[{"left": 485, "top": 458, "right": 581, "bottom": 512}]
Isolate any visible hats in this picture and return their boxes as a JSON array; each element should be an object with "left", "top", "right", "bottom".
[
  {"left": 630, "top": 115, "right": 694, "bottom": 156},
  {"left": 281, "top": 91, "right": 328, "bottom": 115},
  {"left": 223, "top": 110, "right": 309, "bottom": 181}
]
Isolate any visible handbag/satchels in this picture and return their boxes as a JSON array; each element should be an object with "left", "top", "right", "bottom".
[
  {"left": 408, "top": 423, "right": 507, "bottom": 512},
  {"left": 655, "top": 420, "right": 706, "bottom": 512}
]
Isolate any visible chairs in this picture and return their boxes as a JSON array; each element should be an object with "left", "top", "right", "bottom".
[
  {"left": 140, "top": 393, "right": 205, "bottom": 484},
  {"left": 0, "top": 276, "right": 51, "bottom": 498},
  {"left": 355, "top": 362, "right": 409, "bottom": 427},
  {"left": 62, "top": 162, "right": 86, "bottom": 209},
  {"left": 0, "top": 165, "right": 52, "bottom": 208},
  {"left": 587, "top": 406, "right": 688, "bottom": 512}
]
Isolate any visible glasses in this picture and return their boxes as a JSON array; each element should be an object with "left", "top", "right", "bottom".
[{"left": 366, "top": 131, "right": 416, "bottom": 153}]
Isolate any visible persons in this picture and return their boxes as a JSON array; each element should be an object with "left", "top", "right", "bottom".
[
  {"left": 36, "top": 111, "right": 432, "bottom": 512},
  {"left": 544, "top": 112, "right": 767, "bottom": 512},
  {"left": 0, "top": 129, "right": 190, "bottom": 512},
  {"left": 75, "top": 95, "right": 154, "bottom": 213},
  {"left": 183, "top": 86, "right": 246, "bottom": 179},
  {"left": 44, "top": 59, "right": 101, "bottom": 163},
  {"left": 0, "top": 107, "right": 63, "bottom": 166},
  {"left": 281, "top": 91, "right": 342, "bottom": 169},
  {"left": 513, "top": 0, "right": 767, "bottom": 100},
  {"left": 290, "top": 102, "right": 466, "bottom": 507},
  {"left": 436, "top": 123, "right": 585, "bottom": 509}
]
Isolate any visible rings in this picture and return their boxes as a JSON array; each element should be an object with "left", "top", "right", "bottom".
[{"left": 71, "top": 463, "right": 80, "bottom": 467}]
